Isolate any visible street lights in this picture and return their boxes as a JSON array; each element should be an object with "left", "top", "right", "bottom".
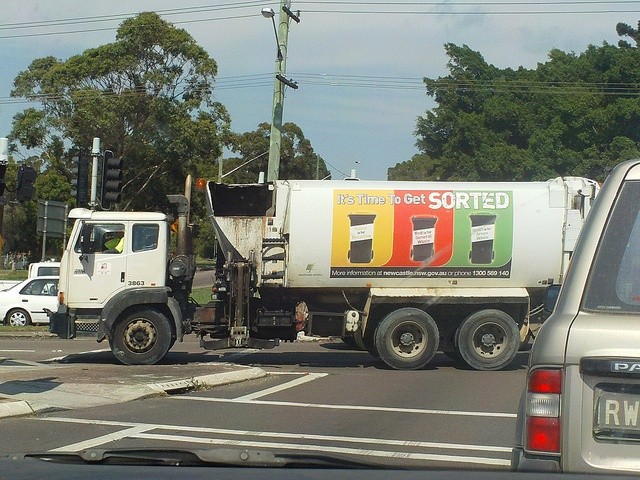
[{"left": 260, "top": 0, "right": 291, "bottom": 179}]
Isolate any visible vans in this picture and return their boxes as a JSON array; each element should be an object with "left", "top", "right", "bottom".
[{"left": 511, "top": 158, "right": 640, "bottom": 477}]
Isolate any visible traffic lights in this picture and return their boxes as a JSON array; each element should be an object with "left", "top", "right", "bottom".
[
  {"left": 70, "top": 149, "right": 90, "bottom": 208},
  {"left": 16, "top": 165, "right": 37, "bottom": 201},
  {"left": 100, "top": 150, "right": 123, "bottom": 210}
]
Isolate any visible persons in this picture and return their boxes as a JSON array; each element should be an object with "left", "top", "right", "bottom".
[{"left": 93, "top": 225, "right": 125, "bottom": 255}]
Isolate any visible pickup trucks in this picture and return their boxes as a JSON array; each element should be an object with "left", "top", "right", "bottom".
[{"left": 0, "top": 258, "right": 61, "bottom": 291}]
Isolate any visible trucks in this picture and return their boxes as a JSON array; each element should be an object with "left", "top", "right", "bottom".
[{"left": 49, "top": 171, "right": 603, "bottom": 371}]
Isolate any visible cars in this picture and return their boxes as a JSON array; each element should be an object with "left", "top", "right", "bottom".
[{"left": 0, "top": 275, "right": 61, "bottom": 327}]
